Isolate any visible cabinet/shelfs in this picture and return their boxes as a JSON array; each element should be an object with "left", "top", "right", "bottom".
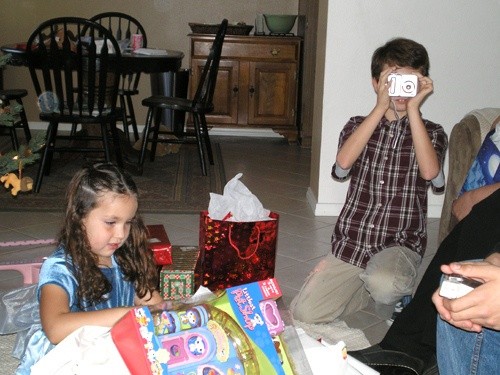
[{"left": 187, "top": 29, "right": 300, "bottom": 143}]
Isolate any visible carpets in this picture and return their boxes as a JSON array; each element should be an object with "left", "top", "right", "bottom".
[{"left": 0, "top": 126, "right": 228, "bottom": 214}]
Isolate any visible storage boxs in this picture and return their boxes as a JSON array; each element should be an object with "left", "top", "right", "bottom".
[
  {"left": 159, "top": 244, "right": 201, "bottom": 301},
  {"left": 146, "top": 221, "right": 173, "bottom": 266}
]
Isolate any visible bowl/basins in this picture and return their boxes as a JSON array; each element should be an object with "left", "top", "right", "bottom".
[{"left": 263, "top": 13, "right": 297, "bottom": 34}]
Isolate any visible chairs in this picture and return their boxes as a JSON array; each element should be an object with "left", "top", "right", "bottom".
[
  {"left": 437, "top": 103, "right": 500, "bottom": 246},
  {"left": 132, "top": 16, "right": 230, "bottom": 178},
  {"left": 68, "top": 10, "right": 152, "bottom": 146},
  {"left": 22, "top": 16, "right": 128, "bottom": 200},
  {"left": 0, "top": 68, "right": 32, "bottom": 153}
]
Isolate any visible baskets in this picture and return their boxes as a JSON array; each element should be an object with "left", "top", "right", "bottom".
[{"left": 189, "top": 23, "right": 254, "bottom": 35}]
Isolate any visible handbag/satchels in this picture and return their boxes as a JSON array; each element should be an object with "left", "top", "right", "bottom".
[
  {"left": 193, "top": 209, "right": 279, "bottom": 292},
  {"left": 208, "top": 172, "right": 272, "bottom": 222}
]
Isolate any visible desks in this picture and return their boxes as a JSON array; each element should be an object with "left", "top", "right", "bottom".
[{"left": 2, "top": 40, "right": 186, "bottom": 180}]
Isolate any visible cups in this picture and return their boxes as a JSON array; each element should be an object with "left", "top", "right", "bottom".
[{"left": 132, "top": 34, "right": 142, "bottom": 51}]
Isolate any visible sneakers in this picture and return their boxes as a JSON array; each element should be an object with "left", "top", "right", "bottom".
[{"left": 386, "top": 294, "right": 412, "bottom": 326}]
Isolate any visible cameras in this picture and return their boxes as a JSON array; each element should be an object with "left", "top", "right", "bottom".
[
  {"left": 438, "top": 271, "right": 482, "bottom": 301},
  {"left": 388, "top": 74, "right": 418, "bottom": 97}
]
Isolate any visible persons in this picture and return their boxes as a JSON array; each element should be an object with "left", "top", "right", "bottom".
[
  {"left": 290, "top": 39, "right": 448, "bottom": 322},
  {"left": 347, "top": 116, "right": 500, "bottom": 375},
  {"left": 15, "top": 162, "right": 165, "bottom": 375}
]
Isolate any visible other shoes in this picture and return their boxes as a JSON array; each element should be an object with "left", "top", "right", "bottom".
[{"left": 348, "top": 343, "right": 439, "bottom": 375}]
